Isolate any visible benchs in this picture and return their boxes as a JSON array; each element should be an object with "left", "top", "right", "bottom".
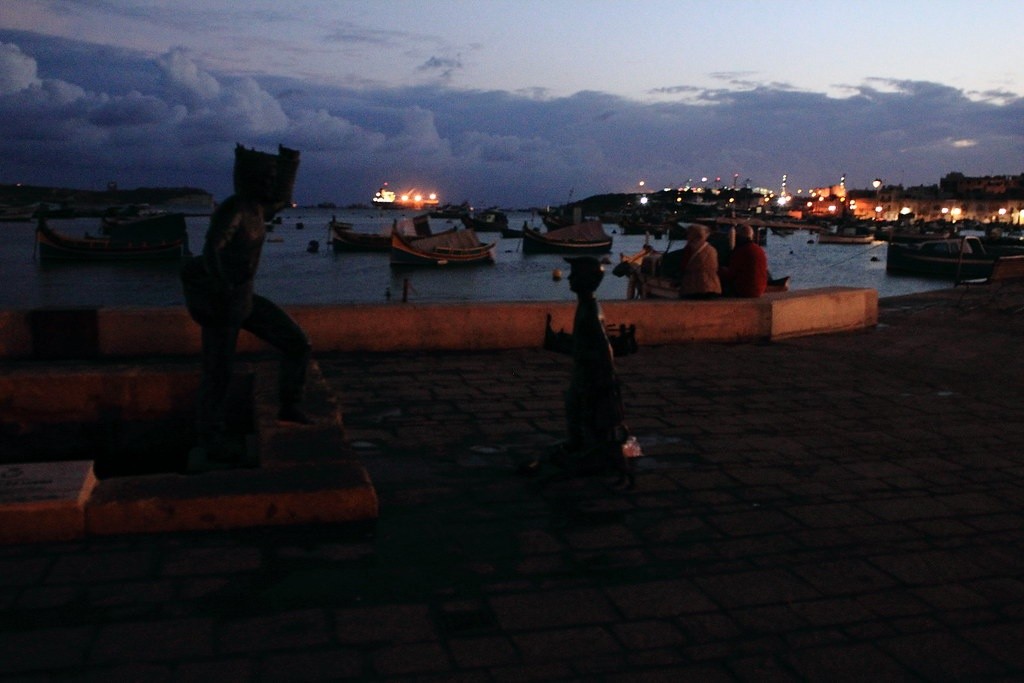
[
  {"left": 961, "top": 255, "right": 1024, "bottom": 312},
  {"left": 1, "top": 286, "right": 877, "bottom": 357}
]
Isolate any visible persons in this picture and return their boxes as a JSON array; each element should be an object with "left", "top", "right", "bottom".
[
  {"left": 170, "top": 169, "right": 315, "bottom": 462},
  {"left": 543, "top": 258, "right": 635, "bottom": 489},
  {"left": 678, "top": 224, "right": 723, "bottom": 302},
  {"left": 727, "top": 224, "right": 767, "bottom": 298}
]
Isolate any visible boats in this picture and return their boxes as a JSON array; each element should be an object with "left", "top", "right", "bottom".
[
  {"left": 329, "top": 213, "right": 432, "bottom": 248},
  {"left": 36, "top": 212, "right": 193, "bottom": 268},
  {"left": 389, "top": 216, "right": 497, "bottom": 264},
  {"left": 618, "top": 213, "right": 950, "bottom": 243},
  {"left": 886, "top": 227, "right": 998, "bottom": 280},
  {"left": 460, "top": 200, "right": 613, "bottom": 252}
]
